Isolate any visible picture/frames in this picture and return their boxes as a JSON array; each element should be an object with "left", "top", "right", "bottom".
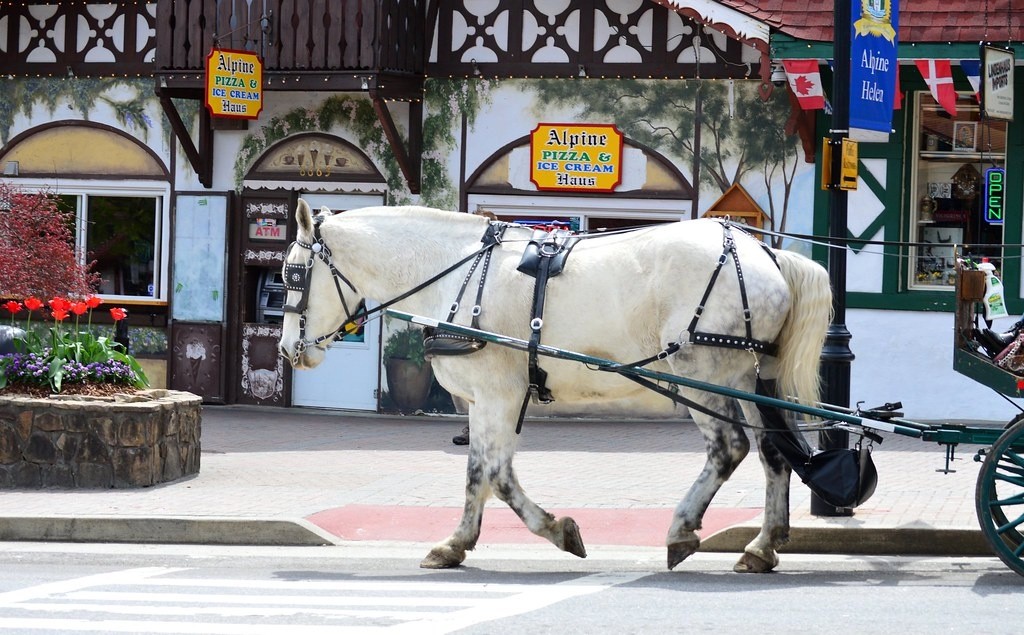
[
  {"left": 952, "top": 120, "right": 978, "bottom": 152},
  {"left": 919, "top": 223, "right": 965, "bottom": 275}
]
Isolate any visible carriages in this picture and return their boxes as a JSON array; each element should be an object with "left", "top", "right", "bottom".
[{"left": 276, "top": 198, "right": 1024, "bottom": 580}]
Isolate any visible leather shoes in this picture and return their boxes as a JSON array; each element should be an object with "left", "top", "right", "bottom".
[{"left": 972, "top": 327, "right": 1016, "bottom": 355}]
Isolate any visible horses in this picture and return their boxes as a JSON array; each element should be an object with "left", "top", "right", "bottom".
[{"left": 274, "top": 198, "right": 839, "bottom": 577}]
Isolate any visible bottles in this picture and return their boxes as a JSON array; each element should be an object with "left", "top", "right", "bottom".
[
  {"left": 975, "top": 257, "right": 996, "bottom": 271},
  {"left": 983, "top": 268, "right": 1009, "bottom": 320}
]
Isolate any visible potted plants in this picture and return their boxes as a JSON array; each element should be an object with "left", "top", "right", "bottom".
[{"left": 383, "top": 328, "right": 435, "bottom": 412}]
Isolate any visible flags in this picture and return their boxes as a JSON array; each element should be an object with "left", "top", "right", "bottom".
[
  {"left": 959, "top": 60, "right": 981, "bottom": 104},
  {"left": 782, "top": 59, "right": 825, "bottom": 110},
  {"left": 914, "top": 59, "right": 958, "bottom": 117}
]
